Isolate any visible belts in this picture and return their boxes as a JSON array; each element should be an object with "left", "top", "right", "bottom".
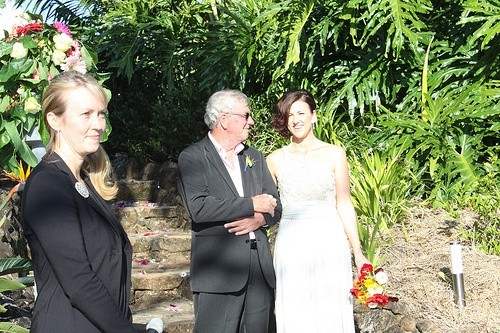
[{"left": 250, "top": 239, "right": 258, "bottom": 249}]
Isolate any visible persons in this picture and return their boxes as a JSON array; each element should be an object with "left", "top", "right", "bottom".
[
  {"left": 21, "top": 70, "right": 165, "bottom": 333},
  {"left": 179, "top": 89, "right": 283, "bottom": 333},
  {"left": 266, "top": 90, "right": 371, "bottom": 333}
]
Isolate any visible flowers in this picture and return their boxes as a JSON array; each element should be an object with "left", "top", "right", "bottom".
[
  {"left": 351, "top": 262, "right": 399, "bottom": 311},
  {"left": 0, "top": 9, "right": 92, "bottom": 116},
  {"left": 244, "top": 154, "right": 258, "bottom": 170}
]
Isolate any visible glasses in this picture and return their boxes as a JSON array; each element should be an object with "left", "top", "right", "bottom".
[{"left": 225, "top": 112, "right": 252, "bottom": 121}]
